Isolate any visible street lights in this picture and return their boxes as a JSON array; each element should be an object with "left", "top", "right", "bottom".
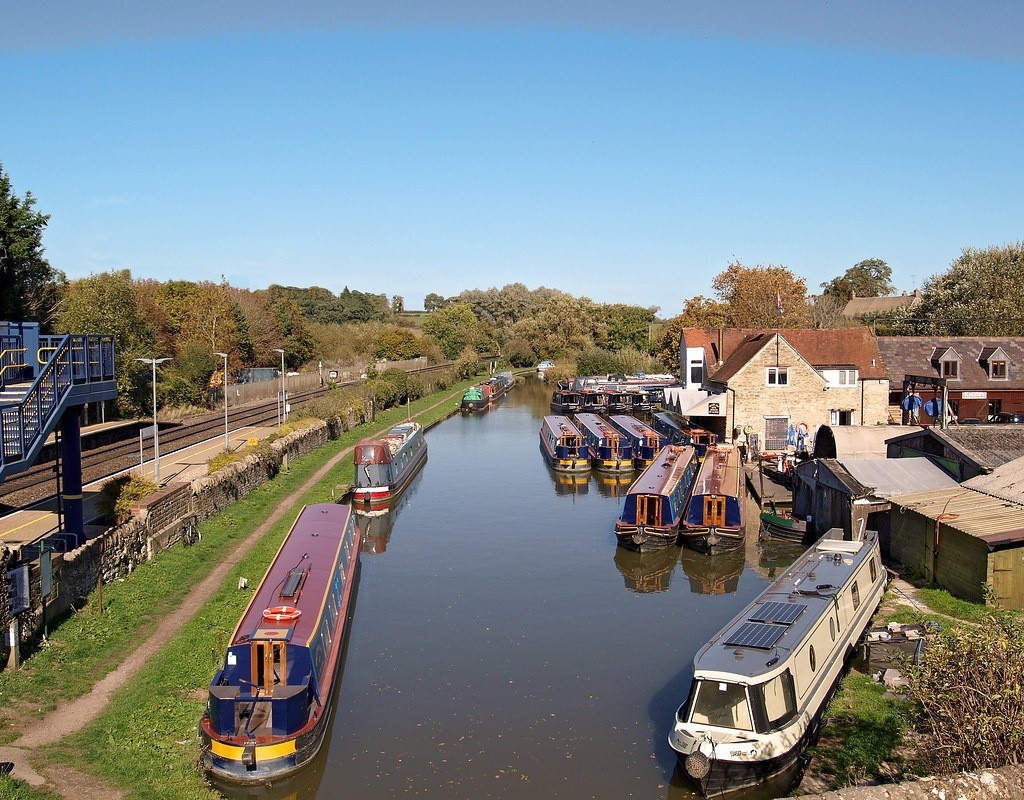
[
  {"left": 132, "top": 358, "right": 177, "bottom": 488},
  {"left": 273, "top": 348, "right": 288, "bottom": 426},
  {"left": 213, "top": 352, "right": 229, "bottom": 452}
]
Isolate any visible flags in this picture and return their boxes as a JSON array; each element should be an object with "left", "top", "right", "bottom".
[{"left": 775, "top": 296, "right": 785, "bottom": 313}]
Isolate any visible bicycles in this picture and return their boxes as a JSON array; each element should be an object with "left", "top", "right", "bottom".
[{"left": 180, "top": 515, "right": 204, "bottom": 550}]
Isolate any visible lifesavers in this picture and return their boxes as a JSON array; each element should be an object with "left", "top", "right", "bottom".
[
  {"left": 643, "top": 430, "right": 654, "bottom": 435},
  {"left": 743, "top": 424, "right": 754, "bottom": 434},
  {"left": 563, "top": 431, "right": 572, "bottom": 434},
  {"left": 603, "top": 430, "right": 613, "bottom": 434},
  {"left": 262, "top": 605, "right": 302, "bottom": 620},
  {"left": 714, "top": 448, "right": 729, "bottom": 453},
  {"left": 796, "top": 421, "right": 810, "bottom": 435}
]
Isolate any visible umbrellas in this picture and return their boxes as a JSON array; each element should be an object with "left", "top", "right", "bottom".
[
  {"left": 786, "top": 424, "right": 796, "bottom": 449},
  {"left": 796, "top": 428, "right": 804, "bottom": 451}
]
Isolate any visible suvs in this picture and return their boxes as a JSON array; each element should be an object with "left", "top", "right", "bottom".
[{"left": 986, "top": 412, "right": 1024, "bottom": 425}]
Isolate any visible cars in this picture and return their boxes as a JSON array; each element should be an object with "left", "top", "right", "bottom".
[{"left": 949, "top": 419, "right": 984, "bottom": 427}]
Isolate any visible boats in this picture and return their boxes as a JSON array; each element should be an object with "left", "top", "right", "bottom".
[
  {"left": 199, "top": 501, "right": 368, "bottom": 784},
  {"left": 614, "top": 445, "right": 699, "bottom": 553},
  {"left": 593, "top": 470, "right": 635, "bottom": 506},
  {"left": 679, "top": 448, "right": 752, "bottom": 556},
  {"left": 614, "top": 543, "right": 680, "bottom": 595},
  {"left": 539, "top": 416, "right": 592, "bottom": 472},
  {"left": 536, "top": 361, "right": 554, "bottom": 373},
  {"left": 551, "top": 471, "right": 590, "bottom": 499},
  {"left": 350, "top": 423, "right": 428, "bottom": 512},
  {"left": 669, "top": 527, "right": 890, "bottom": 793},
  {"left": 678, "top": 540, "right": 747, "bottom": 595},
  {"left": 650, "top": 412, "right": 719, "bottom": 463},
  {"left": 610, "top": 416, "right": 669, "bottom": 472},
  {"left": 549, "top": 374, "right": 678, "bottom": 417},
  {"left": 572, "top": 413, "right": 637, "bottom": 471},
  {"left": 461, "top": 370, "right": 517, "bottom": 415}
]
